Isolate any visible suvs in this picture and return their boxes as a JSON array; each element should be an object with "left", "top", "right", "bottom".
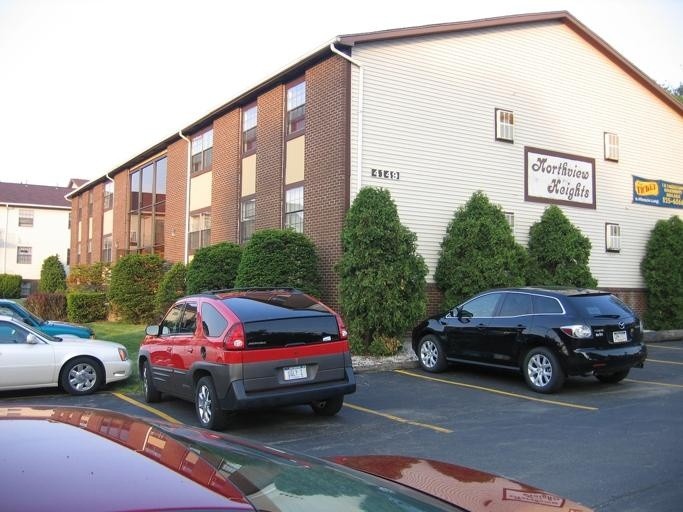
[{"left": 138, "top": 286, "right": 360, "bottom": 431}]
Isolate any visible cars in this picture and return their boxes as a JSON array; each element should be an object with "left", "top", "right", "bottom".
[
  {"left": 0, "top": 404, "right": 595, "bottom": 511},
  {"left": 411, "top": 285, "right": 649, "bottom": 395},
  {"left": 0, "top": 298, "right": 97, "bottom": 342},
  {"left": 0, "top": 314, "right": 134, "bottom": 396}
]
